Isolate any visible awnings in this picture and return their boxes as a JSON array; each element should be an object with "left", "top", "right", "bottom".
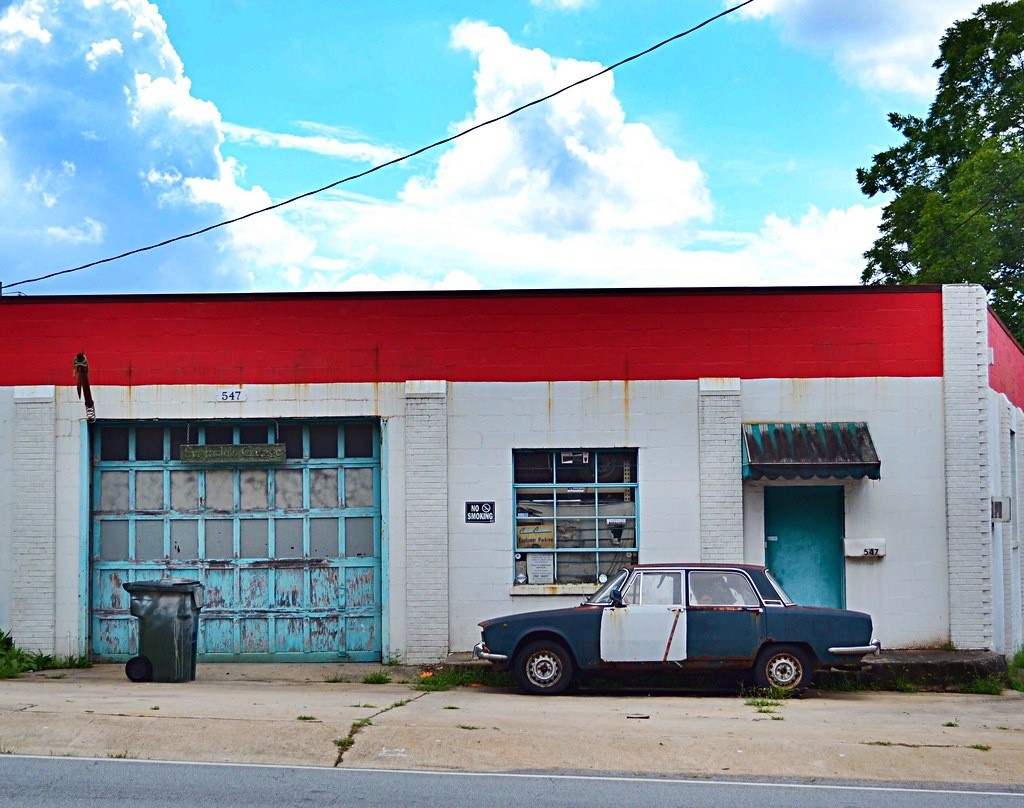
[{"left": 740, "top": 421, "right": 882, "bottom": 482}]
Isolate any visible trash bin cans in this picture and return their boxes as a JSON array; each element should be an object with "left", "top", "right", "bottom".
[{"left": 122, "top": 578, "right": 204, "bottom": 683}]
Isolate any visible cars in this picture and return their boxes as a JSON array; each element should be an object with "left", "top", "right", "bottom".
[{"left": 472, "top": 562, "right": 881, "bottom": 699}]
